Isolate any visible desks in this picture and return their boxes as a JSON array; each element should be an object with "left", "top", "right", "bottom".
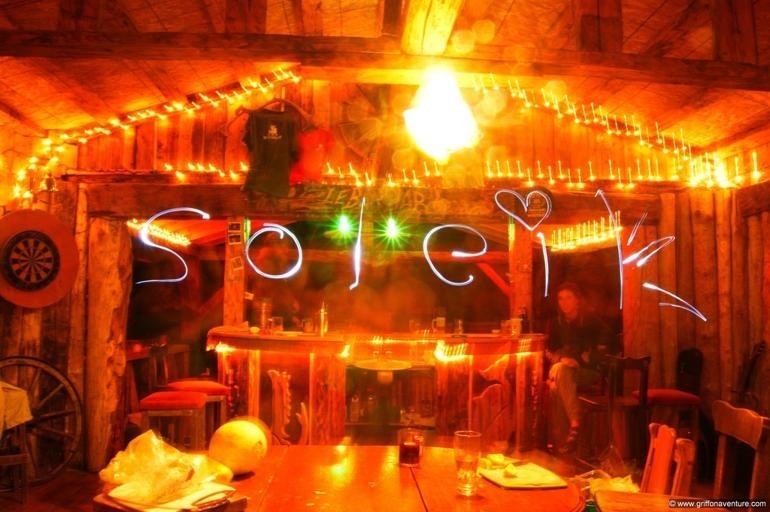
[{"left": 0, "top": 381, "right": 33, "bottom": 504}]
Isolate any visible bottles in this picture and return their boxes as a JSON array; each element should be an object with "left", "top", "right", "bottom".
[
  {"left": 581, "top": 494, "right": 598, "bottom": 512},
  {"left": 520, "top": 306, "right": 529, "bottom": 333}
]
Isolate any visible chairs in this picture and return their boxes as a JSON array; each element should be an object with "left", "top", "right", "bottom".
[{"left": 577, "top": 354, "right": 770, "bottom": 512}]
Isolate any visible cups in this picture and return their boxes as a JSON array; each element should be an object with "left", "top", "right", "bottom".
[
  {"left": 453, "top": 431, "right": 480, "bottom": 496},
  {"left": 268, "top": 316, "right": 283, "bottom": 331},
  {"left": 397, "top": 429, "right": 422, "bottom": 467},
  {"left": 431, "top": 318, "right": 445, "bottom": 333},
  {"left": 509, "top": 318, "right": 521, "bottom": 335},
  {"left": 452, "top": 318, "right": 464, "bottom": 332}
]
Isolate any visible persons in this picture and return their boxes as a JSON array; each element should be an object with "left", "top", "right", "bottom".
[{"left": 543, "top": 283, "right": 611, "bottom": 453}]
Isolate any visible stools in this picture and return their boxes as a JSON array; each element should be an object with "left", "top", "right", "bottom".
[
  {"left": 138, "top": 390, "right": 208, "bottom": 449},
  {"left": 165, "top": 379, "right": 229, "bottom": 434}
]
